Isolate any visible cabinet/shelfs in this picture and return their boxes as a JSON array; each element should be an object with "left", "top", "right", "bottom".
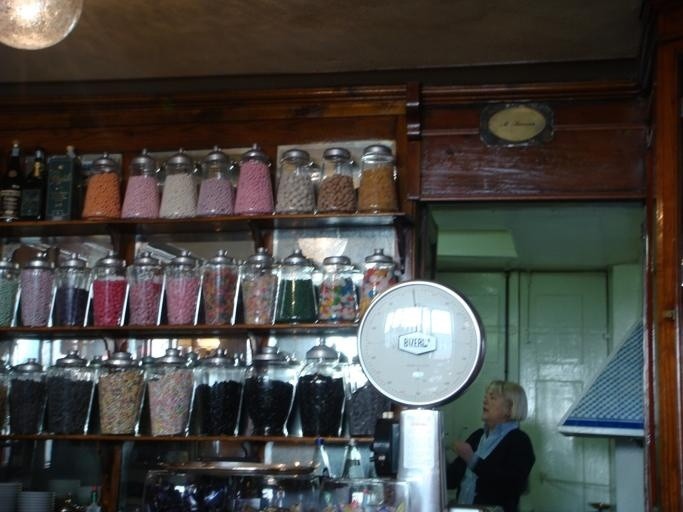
[{"left": 0, "top": 86, "right": 421, "bottom": 511}]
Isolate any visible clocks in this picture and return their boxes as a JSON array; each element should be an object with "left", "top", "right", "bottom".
[{"left": 357, "top": 280, "right": 486, "bottom": 408}]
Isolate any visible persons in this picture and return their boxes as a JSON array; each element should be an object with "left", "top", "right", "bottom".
[{"left": 445, "top": 380, "right": 536, "bottom": 512}]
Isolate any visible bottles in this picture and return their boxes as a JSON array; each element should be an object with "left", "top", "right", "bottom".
[
  {"left": 1, "top": 141, "right": 397, "bottom": 221},
  {"left": 0, "top": 246, "right": 399, "bottom": 328},
  {"left": 0, "top": 339, "right": 393, "bottom": 441}
]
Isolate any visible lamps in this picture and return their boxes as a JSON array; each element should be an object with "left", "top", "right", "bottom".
[{"left": 0, "top": 0, "right": 84, "bottom": 51}]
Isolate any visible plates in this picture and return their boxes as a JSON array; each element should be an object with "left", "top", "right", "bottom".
[{"left": 0, "top": 478, "right": 102, "bottom": 512}]
